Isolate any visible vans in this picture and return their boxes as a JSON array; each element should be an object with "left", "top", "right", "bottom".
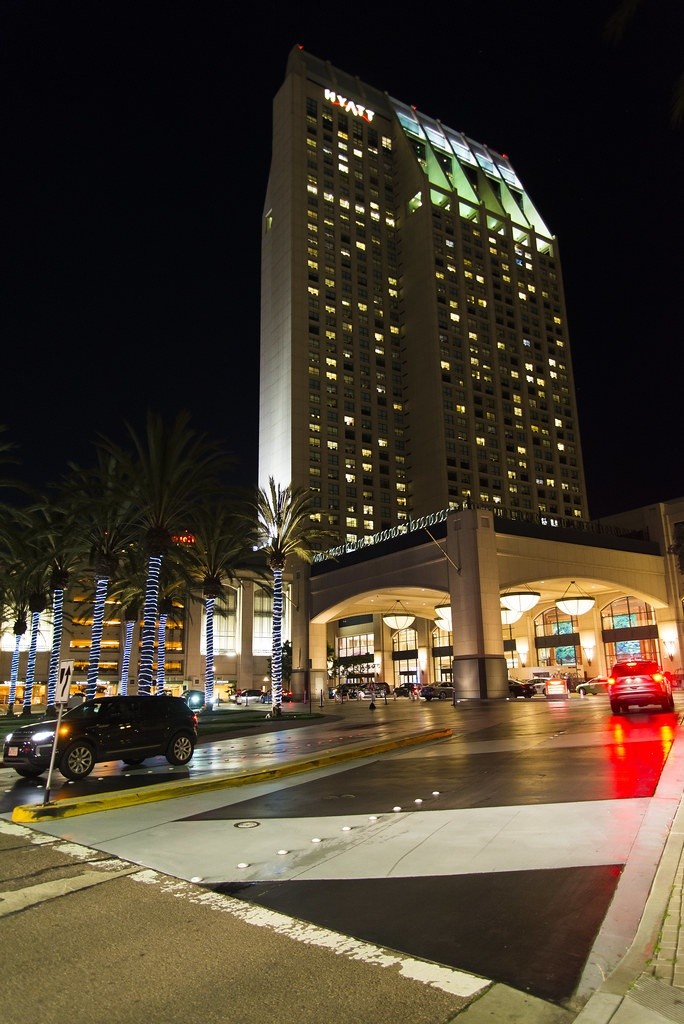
[{"left": 348, "top": 682, "right": 390, "bottom": 699}]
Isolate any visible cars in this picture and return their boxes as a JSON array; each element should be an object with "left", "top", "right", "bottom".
[
  {"left": 508, "top": 679, "right": 538, "bottom": 698},
  {"left": 228, "top": 688, "right": 264, "bottom": 704},
  {"left": 260, "top": 688, "right": 293, "bottom": 704},
  {"left": 328, "top": 683, "right": 360, "bottom": 697},
  {"left": 179, "top": 690, "right": 205, "bottom": 708},
  {"left": 608, "top": 660, "right": 674, "bottom": 713},
  {"left": 54, "top": 693, "right": 85, "bottom": 710},
  {"left": 392, "top": 683, "right": 423, "bottom": 697},
  {"left": 3, "top": 696, "right": 199, "bottom": 780},
  {"left": 526, "top": 677, "right": 552, "bottom": 696},
  {"left": 419, "top": 682, "right": 453, "bottom": 700},
  {"left": 576, "top": 676, "right": 608, "bottom": 695},
  {"left": 77, "top": 683, "right": 107, "bottom": 693}
]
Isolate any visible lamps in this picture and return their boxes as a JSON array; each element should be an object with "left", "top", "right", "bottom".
[
  {"left": 433, "top": 592, "right": 452, "bottom": 632},
  {"left": 382, "top": 600, "right": 417, "bottom": 630},
  {"left": 500, "top": 583, "right": 541, "bottom": 612},
  {"left": 556, "top": 581, "right": 595, "bottom": 615},
  {"left": 502, "top": 608, "right": 523, "bottom": 625}
]
